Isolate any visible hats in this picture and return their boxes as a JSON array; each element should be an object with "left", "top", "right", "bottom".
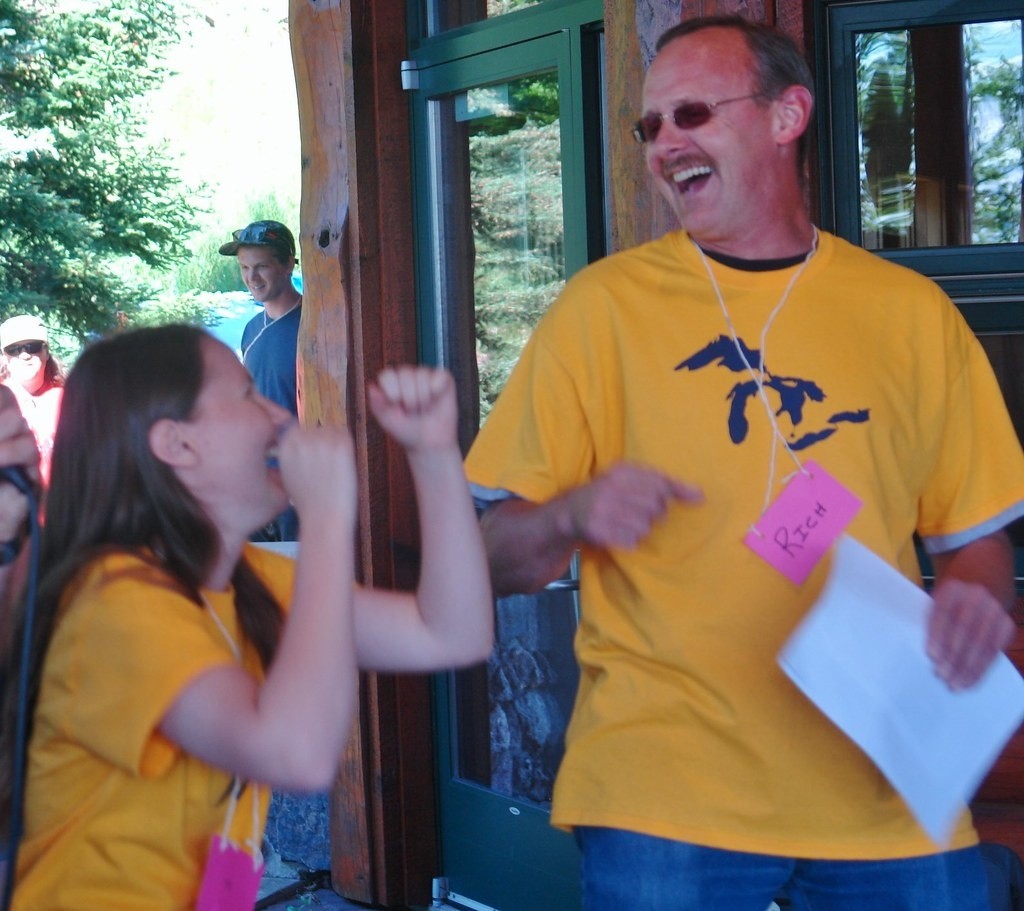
[
  {"left": 0, "top": 315, "right": 48, "bottom": 354},
  {"left": 218, "top": 221, "right": 299, "bottom": 265}
]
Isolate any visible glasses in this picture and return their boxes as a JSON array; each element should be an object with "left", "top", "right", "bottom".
[
  {"left": 631, "top": 90, "right": 767, "bottom": 144},
  {"left": 4, "top": 341, "right": 48, "bottom": 357},
  {"left": 232, "top": 226, "right": 292, "bottom": 254}
]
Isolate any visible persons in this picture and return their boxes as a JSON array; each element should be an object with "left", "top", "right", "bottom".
[
  {"left": 5, "top": 326, "right": 497, "bottom": 911},
  {"left": 217, "top": 220, "right": 304, "bottom": 544},
  {"left": 0, "top": 316, "right": 64, "bottom": 489},
  {"left": 0, "top": 382, "right": 47, "bottom": 597},
  {"left": 464, "top": 12, "right": 1024, "bottom": 911}
]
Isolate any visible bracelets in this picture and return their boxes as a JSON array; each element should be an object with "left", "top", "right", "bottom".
[{"left": 0, "top": 531, "right": 25, "bottom": 567}]
